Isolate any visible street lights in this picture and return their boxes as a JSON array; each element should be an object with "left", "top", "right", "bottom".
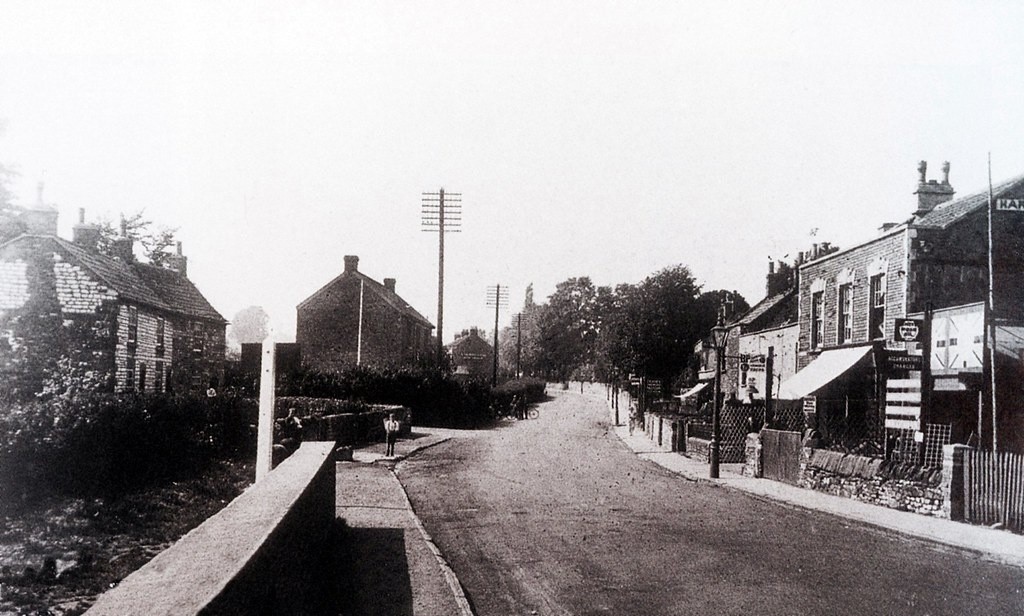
[{"left": 710, "top": 326, "right": 732, "bottom": 479}]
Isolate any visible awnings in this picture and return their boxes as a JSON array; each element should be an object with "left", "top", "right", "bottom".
[
  {"left": 674, "top": 382, "right": 710, "bottom": 398},
  {"left": 771, "top": 345, "right": 873, "bottom": 400}
]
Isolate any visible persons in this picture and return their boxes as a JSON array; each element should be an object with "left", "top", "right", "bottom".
[
  {"left": 384, "top": 413, "right": 399, "bottom": 457},
  {"left": 492, "top": 393, "right": 528, "bottom": 420},
  {"left": 285, "top": 408, "right": 306, "bottom": 446},
  {"left": 627, "top": 406, "right": 638, "bottom": 437}
]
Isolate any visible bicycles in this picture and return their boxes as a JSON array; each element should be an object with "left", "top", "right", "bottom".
[{"left": 506, "top": 402, "right": 539, "bottom": 421}]
